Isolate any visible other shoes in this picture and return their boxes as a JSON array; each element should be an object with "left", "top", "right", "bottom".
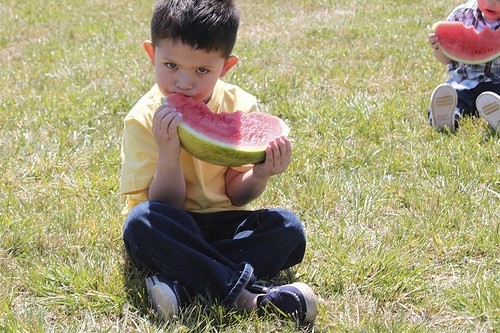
[
  {"left": 475, "top": 90, "right": 500, "bottom": 135},
  {"left": 430, "top": 82, "right": 458, "bottom": 133}
]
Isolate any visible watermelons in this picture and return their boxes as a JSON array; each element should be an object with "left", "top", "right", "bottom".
[
  {"left": 161, "top": 93, "right": 291, "bottom": 166},
  {"left": 432, "top": 21, "right": 500, "bottom": 65}
]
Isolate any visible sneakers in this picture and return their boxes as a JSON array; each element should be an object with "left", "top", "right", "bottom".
[
  {"left": 252, "top": 281, "right": 318, "bottom": 324},
  {"left": 145, "top": 273, "right": 194, "bottom": 325}
]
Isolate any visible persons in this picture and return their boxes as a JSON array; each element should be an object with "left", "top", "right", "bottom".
[
  {"left": 121, "top": 0, "right": 318, "bottom": 323},
  {"left": 427, "top": 0, "right": 500, "bottom": 139}
]
